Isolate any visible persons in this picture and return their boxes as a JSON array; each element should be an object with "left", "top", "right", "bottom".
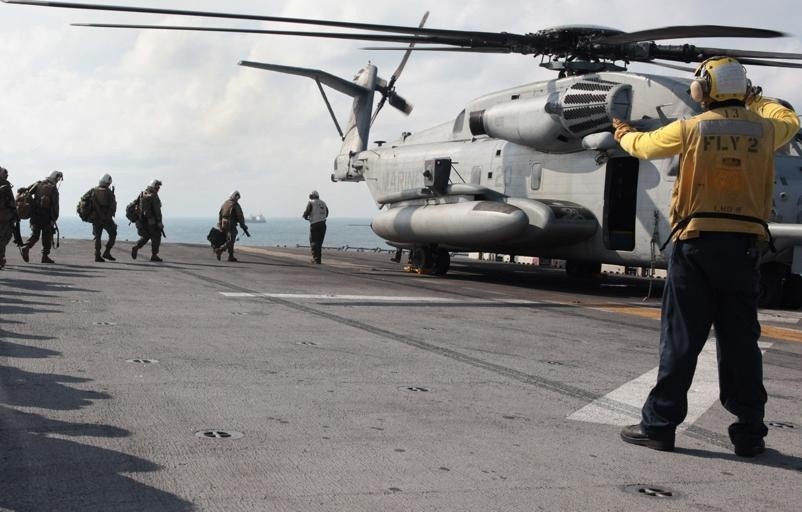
[
  {"left": 132, "top": 180, "right": 164, "bottom": 262},
  {"left": 303, "top": 190, "right": 328, "bottom": 264},
  {"left": 0, "top": 167, "right": 18, "bottom": 269},
  {"left": 19, "top": 172, "right": 63, "bottom": 263},
  {"left": 92, "top": 174, "right": 118, "bottom": 262},
  {"left": 611, "top": 56, "right": 800, "bottom": 457},
  {"left": 215, "top": 190, "right": 248, "bottom": 262}
]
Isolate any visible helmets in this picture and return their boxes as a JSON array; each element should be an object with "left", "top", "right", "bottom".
[
  {"left": 688, "top": 54, "right": 761, "bottom": 108},
  {"left": 227, "top": 189, "right": 242, "bottom": 202},
  {"left": 307, "top": 189, "right": 321, "bottom": 200},
  {"left": 147, "top": 178, "right": 163, "bottom": 193},
  {"left": 46, "top": 169, "right": 64, "bottom": 184},
  {"left": 98, "top": 171, "right": 113, "bottom": 189}
]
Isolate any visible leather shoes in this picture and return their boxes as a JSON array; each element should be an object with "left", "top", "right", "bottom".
[
  {"left": 732, "top": 433, "right": 767, "bottom": 460},
  {"left": 619, "top": 422, "right": 682, "bottom": 451}
]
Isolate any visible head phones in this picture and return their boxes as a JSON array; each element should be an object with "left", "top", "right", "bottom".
[{"left": 687, "top": 56, "right": 752, "bottom": 101}]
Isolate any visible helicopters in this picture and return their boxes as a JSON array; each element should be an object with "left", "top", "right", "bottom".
[{"left": 0, "top": 0, "right": 801, "bottom": 310}]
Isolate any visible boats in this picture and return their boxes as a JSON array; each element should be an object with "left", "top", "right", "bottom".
[{"left": 244, "top": 213, "right": 266, "bottom": 223}]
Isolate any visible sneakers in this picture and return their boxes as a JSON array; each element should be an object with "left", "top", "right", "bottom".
[
  {"left": 149, "top": 255, "right": 162, "bottom": 263},
  {"left": 19, "top": 245, "right": 30, "bottom": 264},
  {"left": 214, "top": 248, "right": 224, "bottom": 263},
  {"left": 310, "top": 258, "right": 323, "bottom": 266},
  {"left": 227, "top": 256, "right": 239, "bottom": 264},
  {"left": 93, "top": 252, "right": 117, "bottom": 264},
  {"left": 130, "top": 245, "right": 139, "bottom": 262},
  {"left": 39, "top": 255, "right": 55, "bottom": 263}
]
